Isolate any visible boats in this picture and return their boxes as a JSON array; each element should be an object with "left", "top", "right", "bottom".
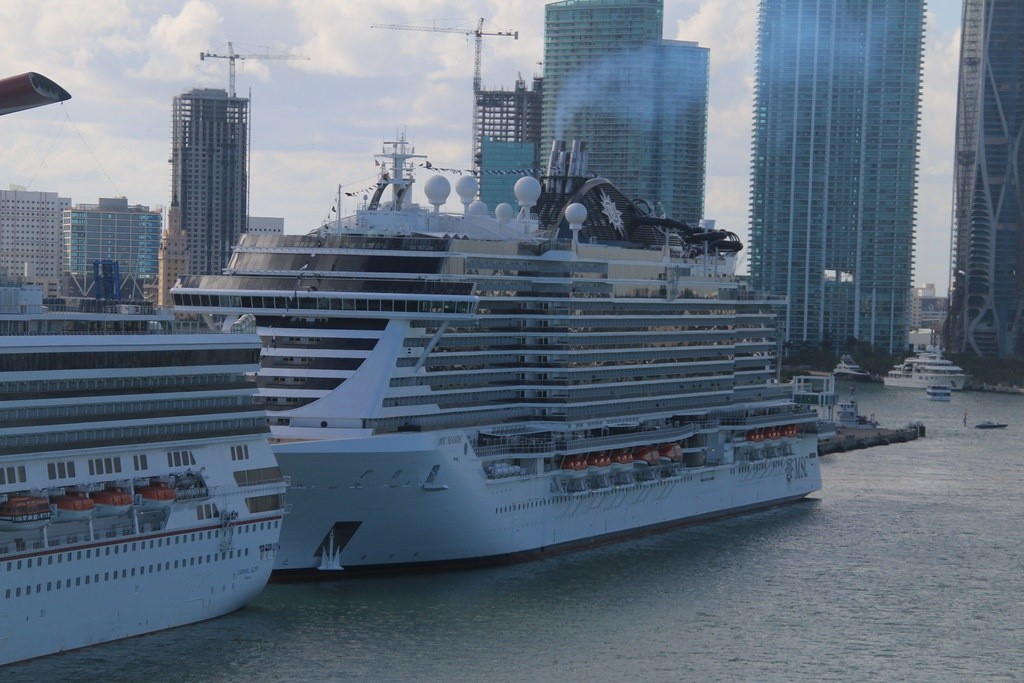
[
  {"left": 0, "top": 496, "right": 51, "bottom": 529},
  {"left": 170, "top": 128, "right": 823, "bottom": 580},
  {"left": 925, "top": 384, "right": 952, "bottom": 401},
  {"left": 884, "top": 344, "right": 973, "bottom": 390},
  {"left": 0, "top": 259, "right": 293, "bottom": 667},
  {"left": 833, "top": 354, "right": 870, "bottom": 378},
  {"left": 136, "top": 486, "right": 177, "bottom": 509},
  {"left": 50, "top": 494, "right": 97, "bottom": 522},
  {"left": 837, "top": 402, "right": 878, "bottom": 429},
  {"left": 92, "top": 490, "right": 134, "bottom": 515},
  {"left": 975, "top": 419, "right": 1008, "bottom": 429}
]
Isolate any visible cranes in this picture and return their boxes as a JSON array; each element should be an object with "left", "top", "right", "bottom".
[
  {"left": 200, "top": 42, "right": 310, "bottom": 98},
  {"left": 370, "top": 16, "right": 519, "bottom": 178}
]
